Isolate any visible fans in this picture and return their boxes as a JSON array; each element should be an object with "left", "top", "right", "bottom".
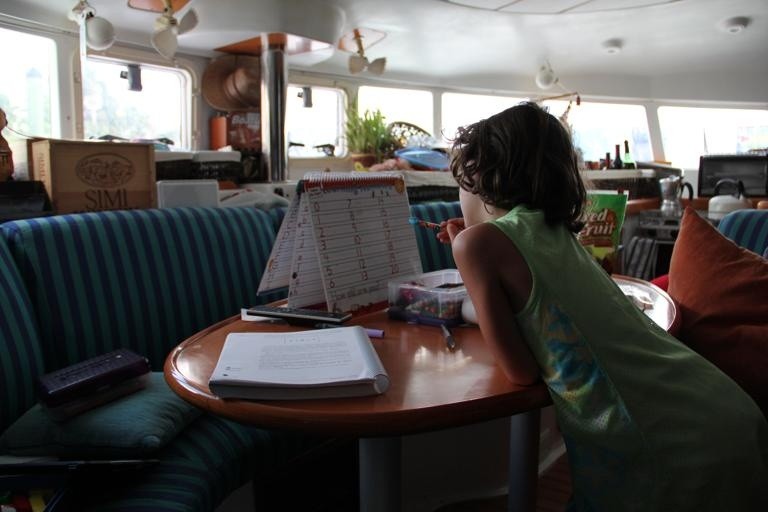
[
  {"left": 151, "top": 0, "right": 198, "bottom": 60},
  {"left": 347, "top": 29, "right": 387, "bottom": 77}
]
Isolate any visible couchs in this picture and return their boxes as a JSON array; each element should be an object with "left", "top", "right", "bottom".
[{"left": 0, "top": 202, "right": 463, "bottom": 510}]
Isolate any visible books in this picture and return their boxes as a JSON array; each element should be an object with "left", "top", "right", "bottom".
[{"left": 208, "top": 327, "right": 390, "bottom": 397}]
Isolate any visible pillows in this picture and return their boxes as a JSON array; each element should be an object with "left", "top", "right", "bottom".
[
  {"left": 668, "top": 205, "right": 767, "bottom": 413},
  {"left": 0, "top": 372, "right": 201, "bottom": 462}
]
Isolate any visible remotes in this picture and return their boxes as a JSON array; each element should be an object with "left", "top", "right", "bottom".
[{"left": 246, "top": 304, "right": 353, "bottom": 324}]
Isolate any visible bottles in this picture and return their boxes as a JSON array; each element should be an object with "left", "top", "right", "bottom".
[
  {"left": 602, "top": 152, "right": 611, "bottom": 169},
  {"left": 611, "top": 145, "right": 623, "bottom": 169},
  {"left": 623, "top": 140, "right": 637, "bottom": 168}
]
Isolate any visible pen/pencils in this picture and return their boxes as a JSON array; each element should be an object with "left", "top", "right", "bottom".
[
  {"left": 387, "top": 294, "right": 462, "bottom": 326},
  {"left": 314, "top": 323, "right": 384, "bottom": 338},
  {"left": 409, "top": 217, "right": 447, "bottom": 231},
  {"left": 441, "top": 323, "right": 455, "bottom": 351}
]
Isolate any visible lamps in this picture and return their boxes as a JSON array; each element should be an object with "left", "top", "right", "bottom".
[{"left": 67, "top": 1, "right": 115, "bottom": 68}]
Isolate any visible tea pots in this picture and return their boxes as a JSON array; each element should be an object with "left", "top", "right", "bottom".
[
  {"left": 707, "top": 178, "right": 753, "bottom": 221},
  {"left": 658, "top": 176, "right": 693, "bottom": 218}
]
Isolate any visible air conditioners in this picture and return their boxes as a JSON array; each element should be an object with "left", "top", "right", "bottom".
[{"left": 535, "top": 59, "right": 555, "bottom": 88}]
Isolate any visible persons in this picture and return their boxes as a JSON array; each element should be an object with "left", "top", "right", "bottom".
[{"left": 438, "top": 103, "right": 767, "bottom": 510}]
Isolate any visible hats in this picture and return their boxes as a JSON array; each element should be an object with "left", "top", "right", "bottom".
[{"left": 202, "top": 54, "right": 259, "bottom": 112}]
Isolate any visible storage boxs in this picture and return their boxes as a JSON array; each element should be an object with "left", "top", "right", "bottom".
[{"left": 27, "top": 139, "right": 159, "bottom": 214}]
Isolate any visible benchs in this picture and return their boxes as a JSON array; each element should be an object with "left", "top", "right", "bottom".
[{"left": 649, "top": 208, "right": 768, "bottom": 416}]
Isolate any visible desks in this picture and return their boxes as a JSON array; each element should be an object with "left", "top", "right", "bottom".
[{"left": 164, "top": 270, "right": 678, "bottom": 511}]
